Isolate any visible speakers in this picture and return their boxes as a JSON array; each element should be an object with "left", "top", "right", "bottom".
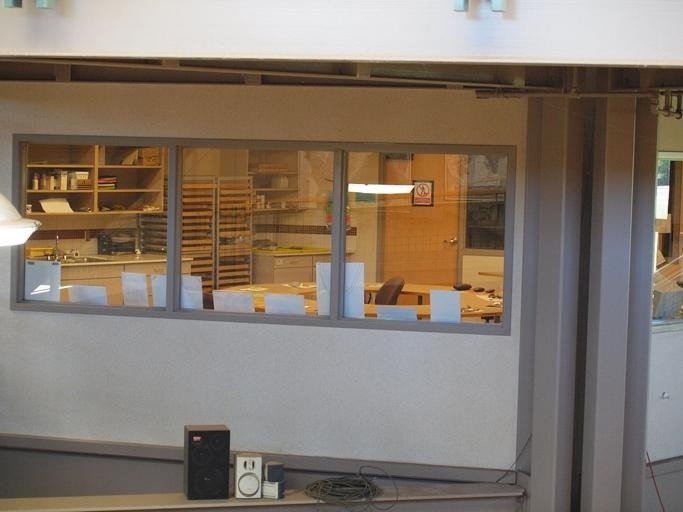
[
  {"left": 183, "top": 424, "right": 230, "bottom": 500},
  {"left": 235, "top": 452, "right": 285, "bottom": 499}
]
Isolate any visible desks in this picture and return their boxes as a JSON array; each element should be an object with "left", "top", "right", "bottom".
[{"left": 221, "top": 282, "right": 503, "bottom": 324}]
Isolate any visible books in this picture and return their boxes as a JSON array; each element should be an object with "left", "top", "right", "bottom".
[{"left": 98, "top": 176, "right": 117, "bottom": 189}]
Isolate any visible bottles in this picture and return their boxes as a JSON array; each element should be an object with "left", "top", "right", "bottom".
[{"left": 31, "top": 171, "right": 39, "bottom": 190}]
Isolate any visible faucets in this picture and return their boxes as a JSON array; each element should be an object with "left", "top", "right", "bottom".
[{"left": 56, "top": 234, "right": 59, "bottom": 251}]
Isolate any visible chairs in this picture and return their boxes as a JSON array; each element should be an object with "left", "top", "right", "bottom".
[{"left": 374, "top": 277, "right": 405, "bottom": 305}]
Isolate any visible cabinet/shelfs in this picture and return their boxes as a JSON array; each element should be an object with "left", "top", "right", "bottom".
[
  {"left": 21, "top": 143, "right": 164, "bottom": 215},
  {"left": 63, "top": 263, "right": 190, "bottom": 307},
  {"left": 219, "top": 148, "right": 316, "bottom": 213},
  {"left": 142, "top": 175, "right": 254, "bottom": 299},
  {"left": 255, "top": 255, "right": 351, "bottom": 287}
]
